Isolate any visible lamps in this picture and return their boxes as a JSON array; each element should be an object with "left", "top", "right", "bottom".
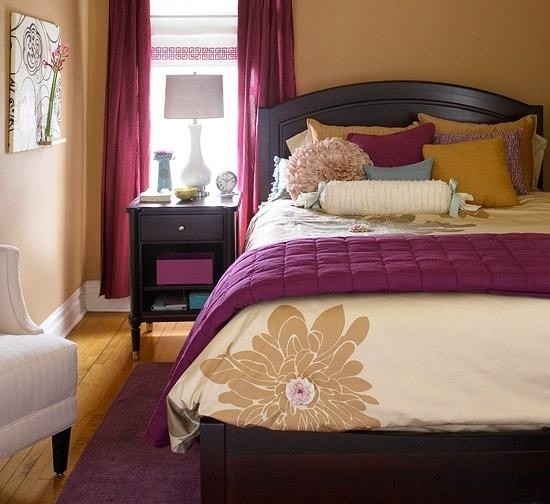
[{"left": 166, "top": 74, "right": 225, "bottom": 188}]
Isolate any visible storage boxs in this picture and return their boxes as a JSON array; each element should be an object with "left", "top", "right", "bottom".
[{"left": 156, "top": 252, "right": 214, "bottom": 285}]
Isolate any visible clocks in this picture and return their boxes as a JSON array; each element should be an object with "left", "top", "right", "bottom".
[{"left": 216, "top": 172, "right": 237, "bottom": 192}]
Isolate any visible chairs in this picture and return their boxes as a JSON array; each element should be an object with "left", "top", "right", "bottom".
[{"left": 1, "top": 247, "right": 76, "bottom": 477}]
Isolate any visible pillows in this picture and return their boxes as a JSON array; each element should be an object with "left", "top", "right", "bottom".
[{"left": 267, "top": 111, "right": 546, "bottom": 216}]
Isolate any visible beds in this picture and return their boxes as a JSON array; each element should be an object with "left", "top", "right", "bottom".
[{"left": 200, "top": 80, "right": 549, "bottom": 504}]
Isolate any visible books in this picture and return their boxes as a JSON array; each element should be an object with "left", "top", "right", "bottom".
[{"left": 140, "top": 192, "right": 172, "bottom": 202}]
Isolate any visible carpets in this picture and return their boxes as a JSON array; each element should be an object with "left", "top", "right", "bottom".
[{"left": 56, "top": 363, "right": 200, "bottom": 504}]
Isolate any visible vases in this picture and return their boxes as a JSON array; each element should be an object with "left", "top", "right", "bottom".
[{"left": 157, "top": 161, "right": 171, "bottom": 193}]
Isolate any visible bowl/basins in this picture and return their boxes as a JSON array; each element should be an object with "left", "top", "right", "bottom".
[{"left": 174, "top": 187, "right": 197, "bottom": 200}]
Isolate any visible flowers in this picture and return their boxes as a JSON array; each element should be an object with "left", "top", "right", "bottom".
[
  {"left": 44, "top": 40, "right": 75, "bottom": 141},
  {"left": 153, "top": 151, "right": 173, "bottom": 161}
]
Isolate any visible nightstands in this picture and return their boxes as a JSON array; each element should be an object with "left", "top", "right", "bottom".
[{"left": 124, "top": 188, "right": 242, "bottom": 361}]
